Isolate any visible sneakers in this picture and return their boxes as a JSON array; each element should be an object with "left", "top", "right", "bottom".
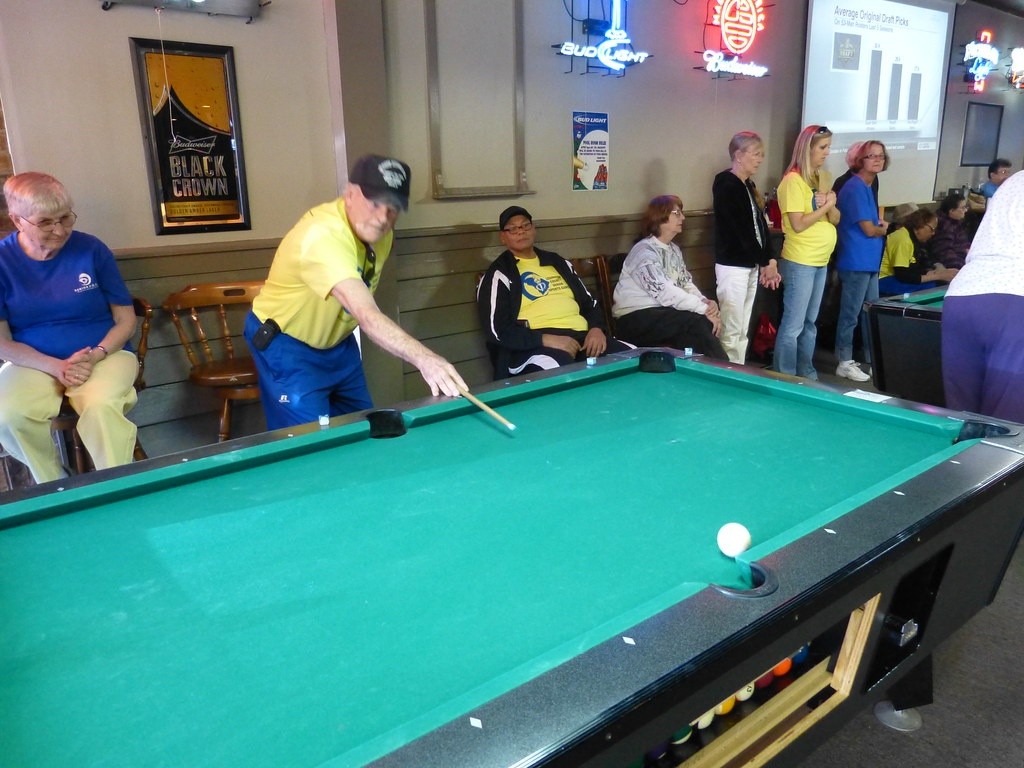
[{"left": 835, "top": 359, "right": 871, "bottom": 382}]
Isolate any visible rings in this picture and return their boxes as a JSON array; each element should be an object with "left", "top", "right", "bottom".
[{"left": 76, "top": 375, "right": 78, "bottom": 379}]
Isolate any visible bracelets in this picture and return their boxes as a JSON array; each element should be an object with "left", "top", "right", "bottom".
[{"left": 97, "top": 346, "right": 107, "bottom": 356}]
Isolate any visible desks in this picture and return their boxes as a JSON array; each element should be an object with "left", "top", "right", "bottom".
[
  {"left": 863, "top": 284, "right": 951, "bottom": 408},
  {"left": 0, "top": 346, "right": 1024, "bottom": 768}
]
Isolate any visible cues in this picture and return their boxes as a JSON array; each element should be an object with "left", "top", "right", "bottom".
[{"left": 455, "top": 384, "right": 517, "bottom": 431}]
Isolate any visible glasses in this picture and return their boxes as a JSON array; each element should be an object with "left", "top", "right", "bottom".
[
  {"left": 814, "top": 127, "right": 828, "bottom": 134},
  {"left": 953, "top": 205, "right": 969, "bottom": 214},
  {"left": 669, "top": 207, "right": 684, "bottom": 218},
  {"left": 20, "top": 212, "right": 78, "bottom": 232},
  {"left": 503, "top": 221, "right": 532, "bottom": 234},
  {"left": 362, "top": 241, "right": 377, "bottom": 285},
  {"left": 925, "top": 223, "right": 937, "bottom": 233},
  {"left": 744, "top": 148, "right": 766, "bottom": 161},
  {"left": 997, "top": 169, "right": 1011, "bottom": 176},
  {"left": 861, "top": 154, "right": 886, "bottom": 160}
]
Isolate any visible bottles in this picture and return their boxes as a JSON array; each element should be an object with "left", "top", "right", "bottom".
[
  {"left": 764, "top": 192, "right": 769, "bottom": 213},
  {"left": 593, "top": 165, "right": 607, "bottom": 190}
]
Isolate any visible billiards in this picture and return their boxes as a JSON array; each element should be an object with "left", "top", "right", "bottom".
[
  {"left": 649, "top": 644, "right": 811, "bottom": 762},
  {"left": 717, "top": 522, "right": 751, "bottom": 558}
]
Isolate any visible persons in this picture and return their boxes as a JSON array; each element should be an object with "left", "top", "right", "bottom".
[
  {"left": 611, "top": 195, "right": 730, "bottom": 362},
  {"left": 773, "top": 125, "right": 841, "bottom": 383},
  {"left": 711, "top": 132, "right": 782, "bottom": 364},
  {"left": 942, "top": 169, "right": 1024, "bottom": 424},
  {"left": 878, "top": 194, "right": 971, "bottom": 296},
  {"left": 242, "top": 154, "right": 469, "bottom": 431},
  {"left": 981, "top": 158, "right": 1010, "bottom": 197},
  {"left": 831, "top": 139, "right": 890, "bottom": 383},
  {"left": 0, "top": 172, "right": 140, "bottom": 484},
  {"left": 476, "top": 206, "right": 638, "bottom": 380}
]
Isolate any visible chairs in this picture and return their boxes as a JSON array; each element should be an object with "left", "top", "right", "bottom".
[
  {"left": 49, "top": 297, "right": 153, "bottom": 474},
  {"left": 597, "top": 252, "right": 628, "bottom": 340},
  {"left": 164, "top": 281, "right": 266, "bottom": 442}
]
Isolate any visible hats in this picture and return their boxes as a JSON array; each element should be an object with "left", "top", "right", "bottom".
[
  {"left": 499, "top": 205, "right": 533, "bottom": 232},
  {"left": 349, "top": 154, "right": 410, "bottom": 214}
]
